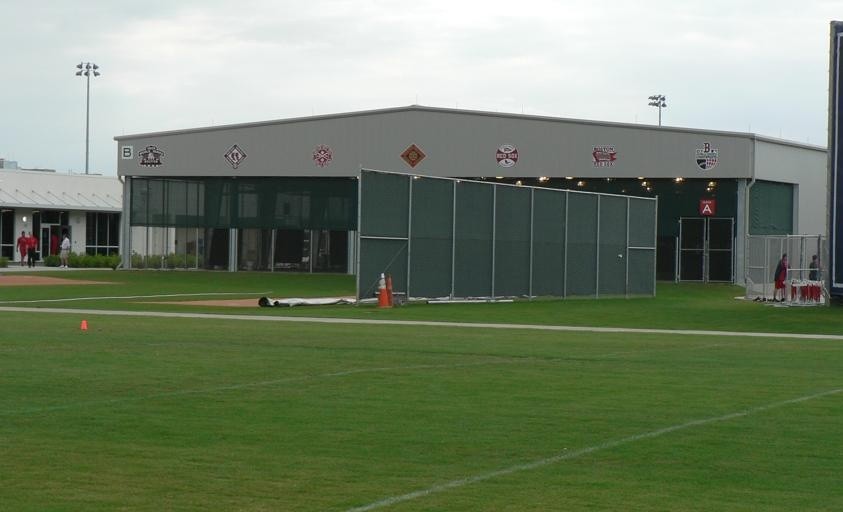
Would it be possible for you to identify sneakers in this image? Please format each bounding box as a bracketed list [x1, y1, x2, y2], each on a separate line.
[60, 265, 68, 269]
[772, 299, 780, 302]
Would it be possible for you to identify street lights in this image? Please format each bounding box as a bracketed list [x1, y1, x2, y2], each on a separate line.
[75, 62, 100, 174]
[648, 94, 666, 126]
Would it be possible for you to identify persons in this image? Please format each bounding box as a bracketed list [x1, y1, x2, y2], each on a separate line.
[15, 231, 27, 266]
[808, 255, 819, 280]
[24, 232, 37, 268]
[58, 234, 70, 269]
[773, 254, 792, 302]
[50, 232, 59, 256]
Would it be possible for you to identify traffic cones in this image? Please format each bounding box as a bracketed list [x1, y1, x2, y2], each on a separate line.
[376, 272, 394, 308]
